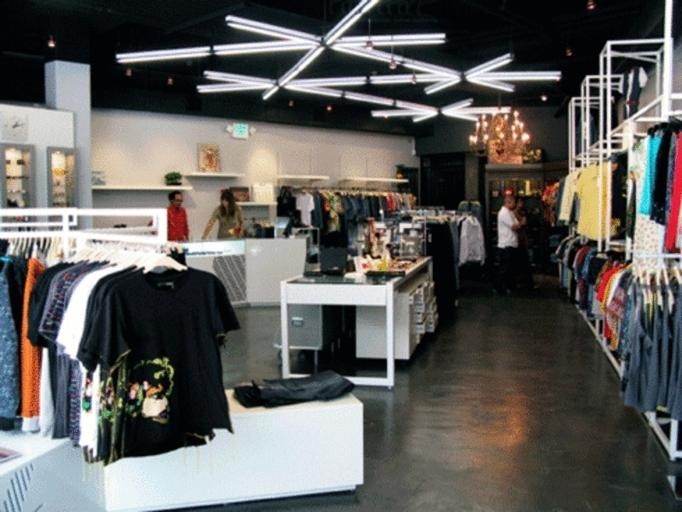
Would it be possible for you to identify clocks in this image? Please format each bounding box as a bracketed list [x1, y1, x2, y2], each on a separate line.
[232, 122, 249, 139]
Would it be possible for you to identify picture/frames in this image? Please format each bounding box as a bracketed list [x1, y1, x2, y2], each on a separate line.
[196, 142, 222, 174]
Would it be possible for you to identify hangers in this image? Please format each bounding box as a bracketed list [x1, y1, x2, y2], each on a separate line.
[2, 232, 188, 276]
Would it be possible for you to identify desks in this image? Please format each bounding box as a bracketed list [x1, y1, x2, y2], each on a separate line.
[278, 255, 433, 390]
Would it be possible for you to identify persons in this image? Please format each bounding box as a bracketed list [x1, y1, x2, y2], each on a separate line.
[492, 195, 538, 298]
[146, 191, 189, 242]
[511, 198, 536, 287]
[201, 189, 245, 241]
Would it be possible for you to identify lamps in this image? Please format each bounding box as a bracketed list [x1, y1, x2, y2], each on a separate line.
[468, 91, 542, 164]
[116, 0, 564, 124]
